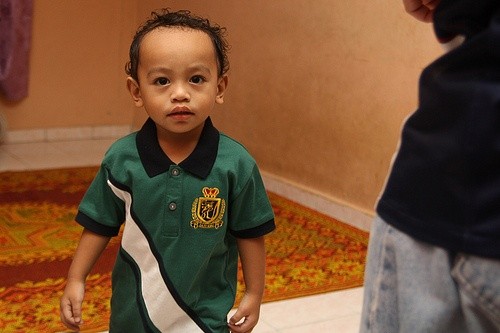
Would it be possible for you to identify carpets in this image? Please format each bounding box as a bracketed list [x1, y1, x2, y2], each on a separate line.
[0, 165, 370, 333]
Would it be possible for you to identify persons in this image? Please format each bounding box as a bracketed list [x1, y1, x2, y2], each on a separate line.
[60, 8, 277, 333]
[358, 0, 500, 333]
